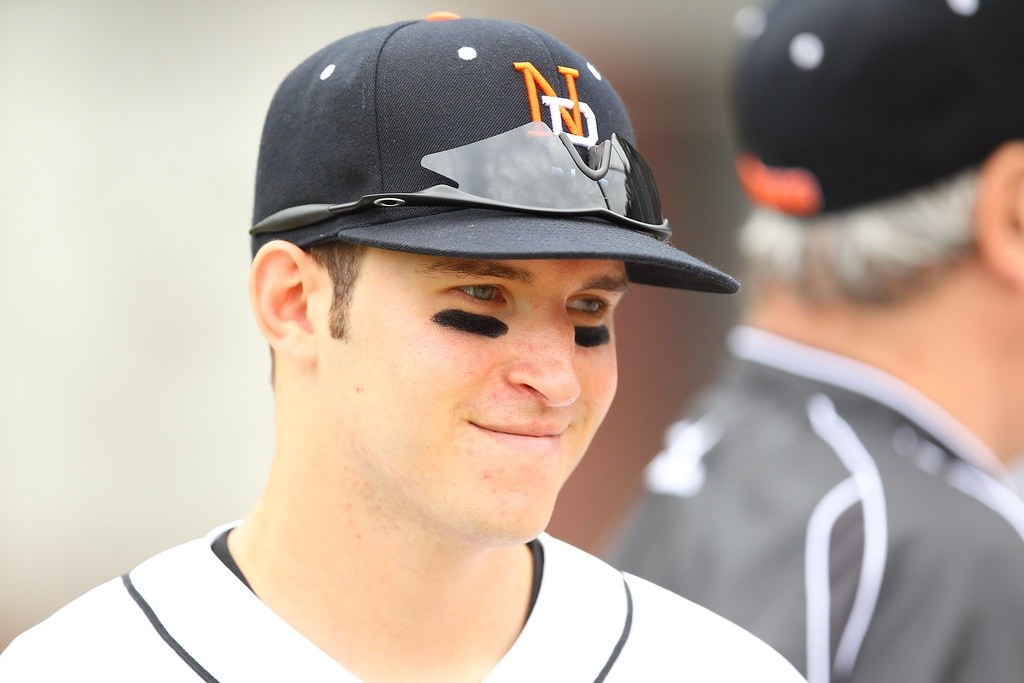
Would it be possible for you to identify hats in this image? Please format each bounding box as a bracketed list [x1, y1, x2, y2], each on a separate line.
[250, 11, 739, 293]
[729, 0, 1024, 221]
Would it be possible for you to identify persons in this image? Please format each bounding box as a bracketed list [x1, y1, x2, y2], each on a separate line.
[0, 13, 807, 683]
[607, 0, 1024, 683]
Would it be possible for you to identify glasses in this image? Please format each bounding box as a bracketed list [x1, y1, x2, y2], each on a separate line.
[249, 121, 673, 239]
